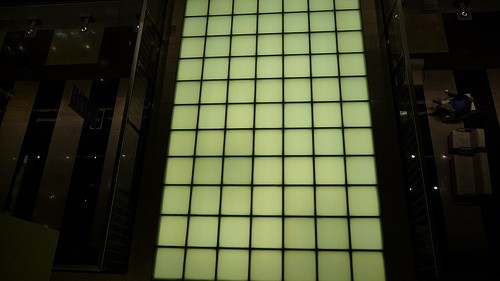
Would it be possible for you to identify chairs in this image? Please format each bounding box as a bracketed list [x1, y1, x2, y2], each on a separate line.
[403, 59, 425, 86]
[449, 128, 486, 153]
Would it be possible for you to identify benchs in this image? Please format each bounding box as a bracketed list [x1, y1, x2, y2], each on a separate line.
[451, 153, 492, 196]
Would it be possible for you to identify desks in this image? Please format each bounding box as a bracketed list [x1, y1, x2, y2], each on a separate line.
[392, 13, 448, 53]
